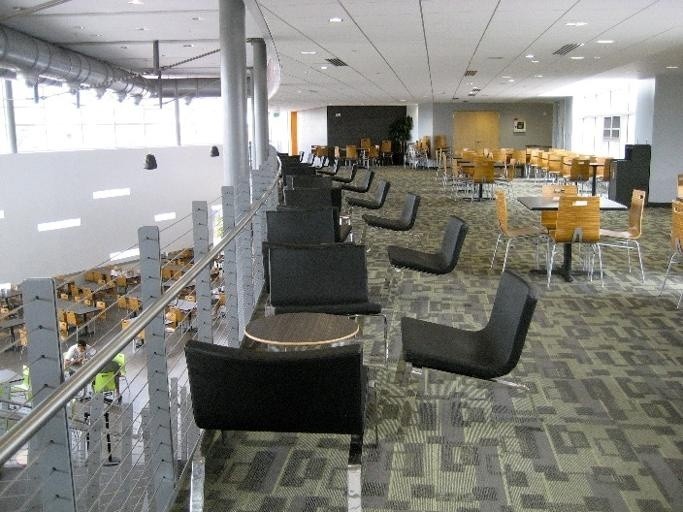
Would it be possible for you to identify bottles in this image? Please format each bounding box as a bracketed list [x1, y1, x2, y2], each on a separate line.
[552, 188, 562, 200]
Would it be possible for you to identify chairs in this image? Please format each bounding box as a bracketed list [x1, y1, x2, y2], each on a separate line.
[0, 249, 225, 404]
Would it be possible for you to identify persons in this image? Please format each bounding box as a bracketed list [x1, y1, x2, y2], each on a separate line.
[64, 338, 97, 366]
